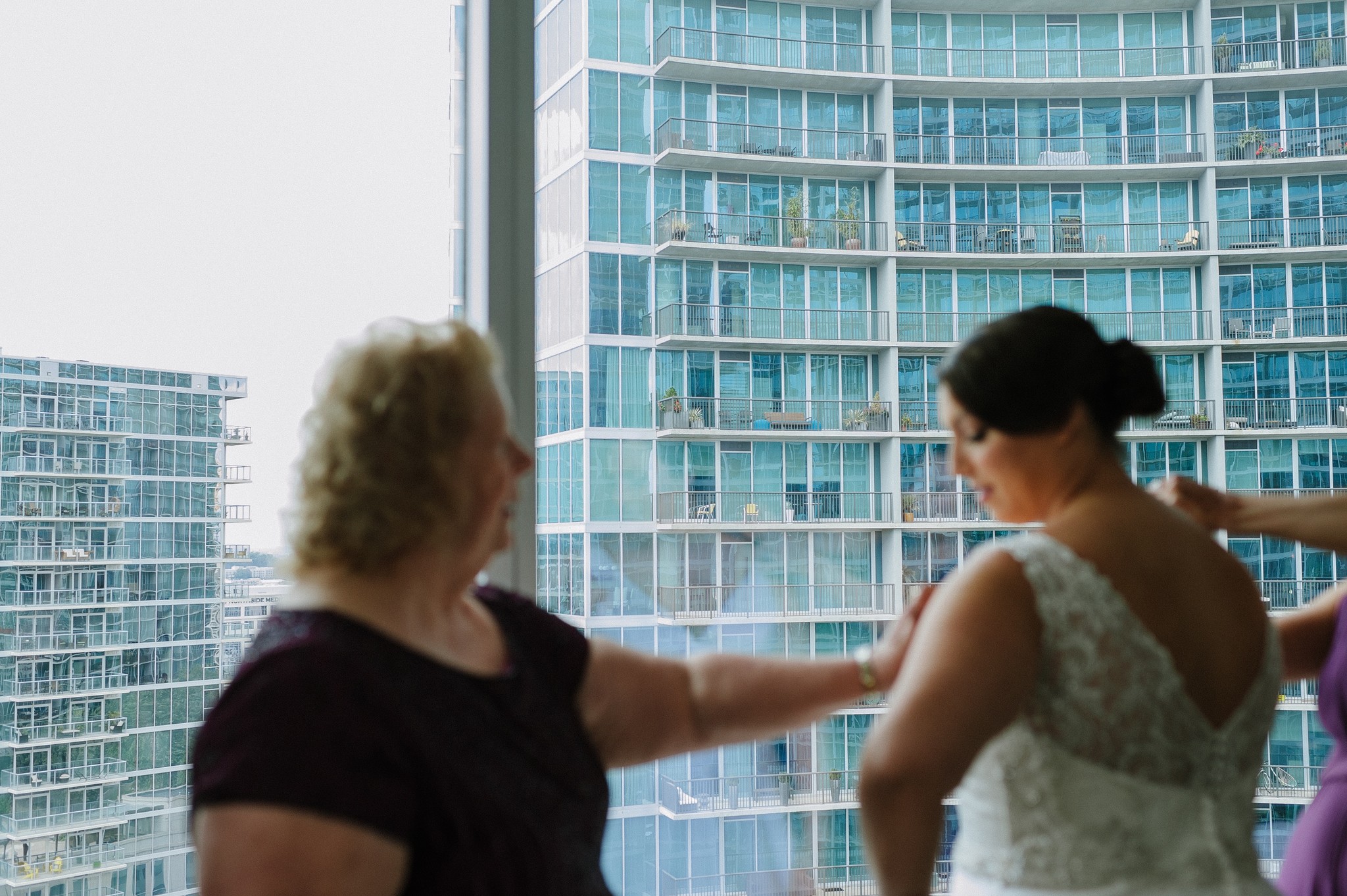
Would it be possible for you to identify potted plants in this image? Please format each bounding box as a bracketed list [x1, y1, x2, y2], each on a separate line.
[725, 768, 841, 809]
[786, 188, 862, 249]
[843, 390, 919, 522]
[232, 428, 249, 440]
[1061, 217, 1081, 243]
[660, 218, 695, 241]
[902, 494, 916, 521]
[1236, 125, 1267, 159]
[786, 502, 795, 522]
[688, 406, 706, 429]
[1190, 408, 1210, 429]
[1215, 32, 1332, 73]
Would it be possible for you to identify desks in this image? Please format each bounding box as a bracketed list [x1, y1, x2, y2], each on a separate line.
[918, 245, 928, 251]
[997, 230, 1014, 252]
[804, 503, 823, 524]
[1159, 243, 1173, 250]
[764, 149, 775, 155]
[22, 642, 32, 651]
[1304, 145, 1319, 156]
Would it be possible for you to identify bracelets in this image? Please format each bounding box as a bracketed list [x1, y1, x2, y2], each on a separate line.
[858, 646, 878, 696]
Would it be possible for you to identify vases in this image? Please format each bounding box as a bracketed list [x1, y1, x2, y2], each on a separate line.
[664, 412, 690, 429]
[1262, 154, 1271, 159]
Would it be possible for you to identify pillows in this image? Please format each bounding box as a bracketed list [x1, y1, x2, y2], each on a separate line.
[1158, 411, 1190, 420]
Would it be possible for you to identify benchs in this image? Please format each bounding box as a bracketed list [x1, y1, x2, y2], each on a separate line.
[663, 781, 697, 812]
[1227, 241, 1279, 249]
[764, 412, 812, 429]
[1151, 410, 1191, 429]
[1250, 422, 1297, 429]
[912, 423, 927, 432]
[1237, 60, 1276, 72]
[1226, 417, 1247, 430]
[753, 788, 794, 802]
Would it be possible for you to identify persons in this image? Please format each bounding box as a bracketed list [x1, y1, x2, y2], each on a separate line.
[854, 306, 1282, 896]
[189, 324, 933, 896]
[1160, 474, 1347, 896]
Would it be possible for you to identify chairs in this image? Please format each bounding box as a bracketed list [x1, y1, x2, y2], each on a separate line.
[718, 411, 754, 430]
[689, 503, 759, 523]
[1253, 331, 1272, 339]
[15, 461, 247, 878]
[703, 222, 763, 245]
[1227, 138, 1342, 339]
[739, 143, 795, 157]
[896, 226, 1199, 252]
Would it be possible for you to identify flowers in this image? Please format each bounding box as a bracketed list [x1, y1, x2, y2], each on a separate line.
[1256, 142, 1284, 154]
[657, 400, 681, 414]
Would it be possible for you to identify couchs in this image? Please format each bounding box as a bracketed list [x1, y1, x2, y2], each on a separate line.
[746, 870, 816, 896]
